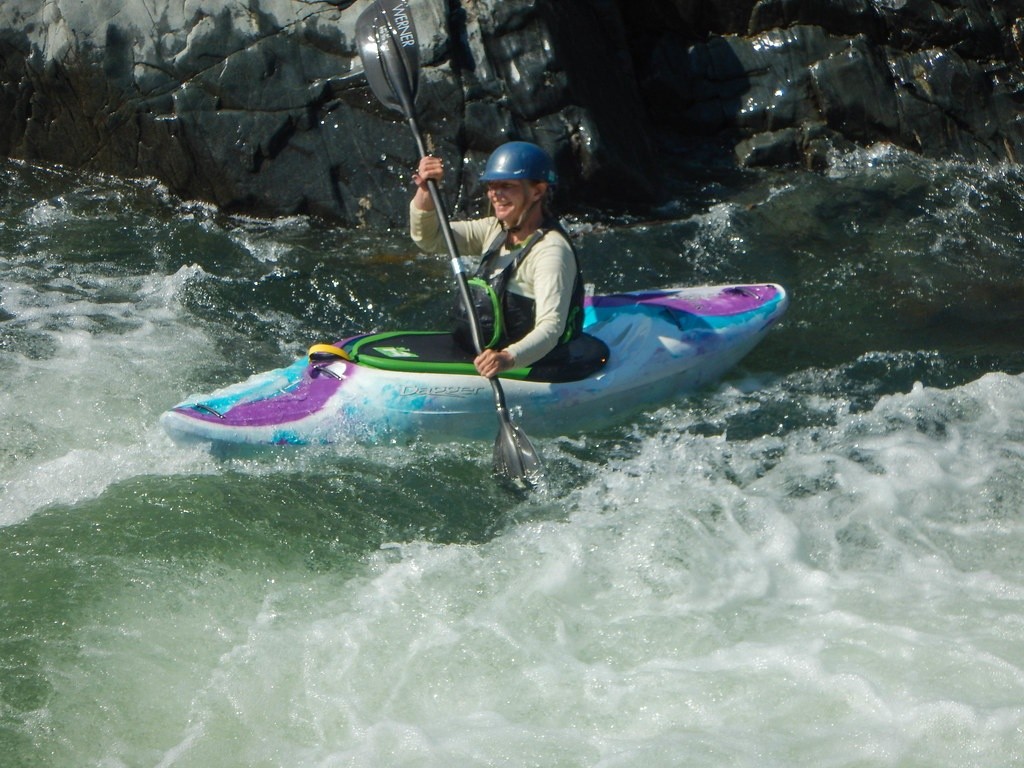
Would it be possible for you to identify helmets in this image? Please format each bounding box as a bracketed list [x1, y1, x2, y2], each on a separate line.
[478, 141, 556, 184]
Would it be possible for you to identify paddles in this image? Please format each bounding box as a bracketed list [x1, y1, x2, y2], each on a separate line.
[358, 0, 550, 501]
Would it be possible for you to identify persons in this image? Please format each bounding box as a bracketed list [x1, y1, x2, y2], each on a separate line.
[410, 142, 585, 378]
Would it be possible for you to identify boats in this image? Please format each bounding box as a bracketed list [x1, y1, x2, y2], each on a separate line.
[155, 284, 792, 457]
